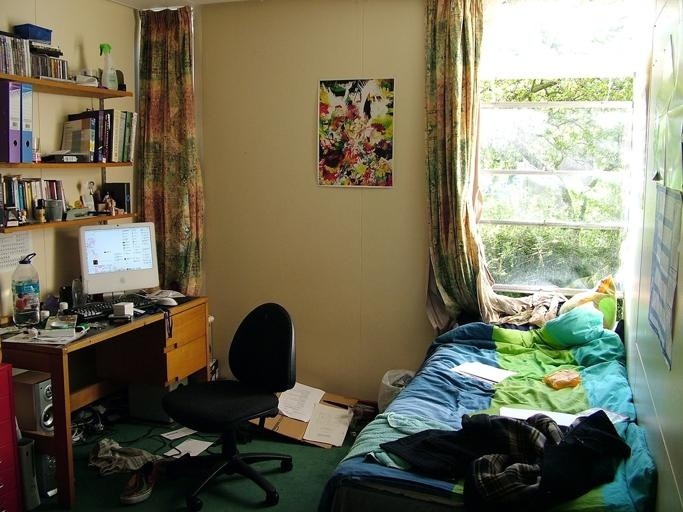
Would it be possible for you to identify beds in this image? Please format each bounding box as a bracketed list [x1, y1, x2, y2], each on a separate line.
[320, 293, 661, 512]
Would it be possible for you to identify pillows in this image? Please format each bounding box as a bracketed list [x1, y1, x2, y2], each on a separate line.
[558, 275, 617, 330]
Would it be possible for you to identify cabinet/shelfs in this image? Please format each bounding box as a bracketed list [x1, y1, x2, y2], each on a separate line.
[0, 75, 137, 233]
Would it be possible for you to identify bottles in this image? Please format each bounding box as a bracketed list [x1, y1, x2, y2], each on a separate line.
[35, 199, 47, 223]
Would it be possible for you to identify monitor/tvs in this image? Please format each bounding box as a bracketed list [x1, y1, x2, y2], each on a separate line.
[78, 222, 160, 299]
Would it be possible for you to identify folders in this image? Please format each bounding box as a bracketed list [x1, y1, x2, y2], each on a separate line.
[0, 81, 33, 164]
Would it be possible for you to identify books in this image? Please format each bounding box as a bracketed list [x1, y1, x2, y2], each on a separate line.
[0, 171, 67, 220]
[59, 109, 139, 163]
[101, 182, 127, 214]
[0, 33, 77, 83]
[44, 314, 78, 336]
[125, 182, 130, 214]
[40, 153, 89, 164]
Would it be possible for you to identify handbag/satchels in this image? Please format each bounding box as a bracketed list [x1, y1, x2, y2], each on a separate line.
[88, 437, 164, 477]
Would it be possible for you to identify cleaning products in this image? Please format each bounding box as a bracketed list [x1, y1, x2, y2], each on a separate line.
[100, 44, 119, 90]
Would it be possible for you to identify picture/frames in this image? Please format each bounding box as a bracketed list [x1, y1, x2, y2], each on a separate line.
[317, 78, 396, 189]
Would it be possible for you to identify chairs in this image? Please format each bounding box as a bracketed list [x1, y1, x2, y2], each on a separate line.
[163, 301, 296, 512]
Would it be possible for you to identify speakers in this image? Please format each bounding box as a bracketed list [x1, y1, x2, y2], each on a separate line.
[11, 369, 55, 433]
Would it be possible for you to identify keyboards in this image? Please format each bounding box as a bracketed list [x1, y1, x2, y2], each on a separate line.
[63, 294, 155, 321]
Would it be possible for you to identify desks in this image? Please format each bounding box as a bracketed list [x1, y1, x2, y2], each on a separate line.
[0, 297, 209, 512]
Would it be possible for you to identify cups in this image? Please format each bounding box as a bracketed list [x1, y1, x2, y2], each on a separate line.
[48, 199, 63, 221]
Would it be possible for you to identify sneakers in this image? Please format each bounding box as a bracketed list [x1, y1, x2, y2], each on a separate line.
[119, 461, 157, 505]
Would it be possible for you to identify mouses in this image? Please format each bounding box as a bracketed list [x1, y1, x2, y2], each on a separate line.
[156, 297, 178, 307]
